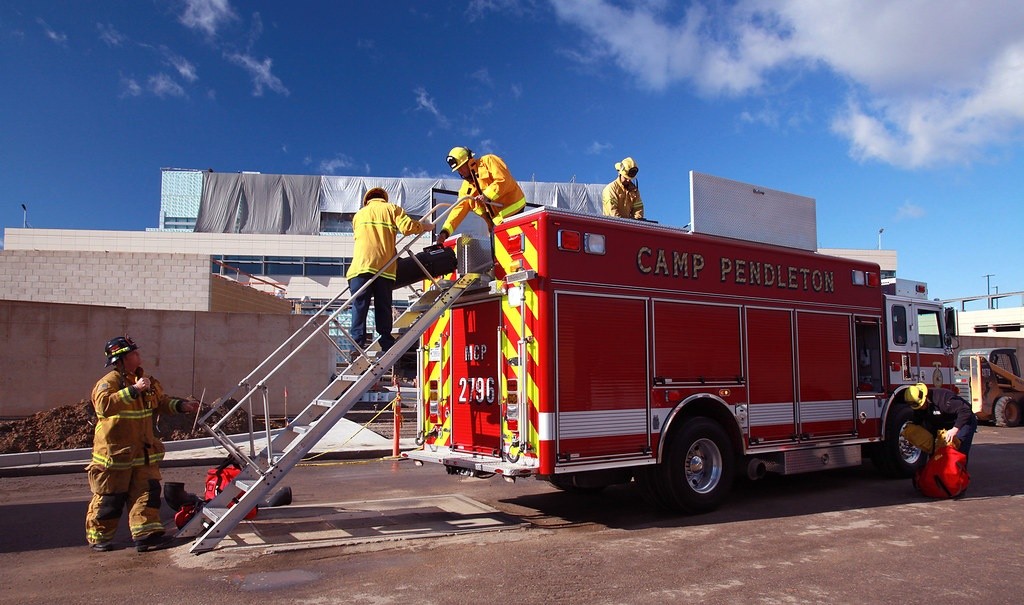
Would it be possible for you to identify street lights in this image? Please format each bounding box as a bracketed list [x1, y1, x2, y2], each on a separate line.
[982, 274, 995, 309]
[878, 227, 884, 250]
[21, 203, 27, 228]
[991, 286, 998, 309]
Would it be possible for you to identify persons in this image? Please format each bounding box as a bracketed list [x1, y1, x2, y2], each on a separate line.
[84, 337, 201, 552]
[348, 187, 436, 358]
[435, 145, 528, 226]
[603, 156, 644, 221]
[906, 384, 977, 497]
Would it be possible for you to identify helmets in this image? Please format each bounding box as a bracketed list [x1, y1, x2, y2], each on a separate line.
[614, 157, 638, 180]
[445, 146, 475, 173]
[103, 332, 142, 368]
[363, 187, 389, 206]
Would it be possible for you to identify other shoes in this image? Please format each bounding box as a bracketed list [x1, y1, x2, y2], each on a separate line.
[348, 350, 359, 363]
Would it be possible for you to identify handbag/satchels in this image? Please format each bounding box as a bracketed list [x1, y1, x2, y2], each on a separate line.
[918, 444, 970, 500]
[391, 243, 458, 290]
[204, 452, 258, 520]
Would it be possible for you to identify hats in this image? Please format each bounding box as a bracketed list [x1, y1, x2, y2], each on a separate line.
[905, 382, 928, 410]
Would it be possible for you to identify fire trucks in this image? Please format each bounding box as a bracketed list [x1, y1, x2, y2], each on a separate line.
[402, 171, 960, 517]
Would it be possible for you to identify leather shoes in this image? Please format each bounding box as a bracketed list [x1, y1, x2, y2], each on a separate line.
[134, 531, 173, 552]
[88, 541, 114, 551]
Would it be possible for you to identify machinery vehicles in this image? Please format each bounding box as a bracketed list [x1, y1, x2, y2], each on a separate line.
[955, 347, 1024, 427]
